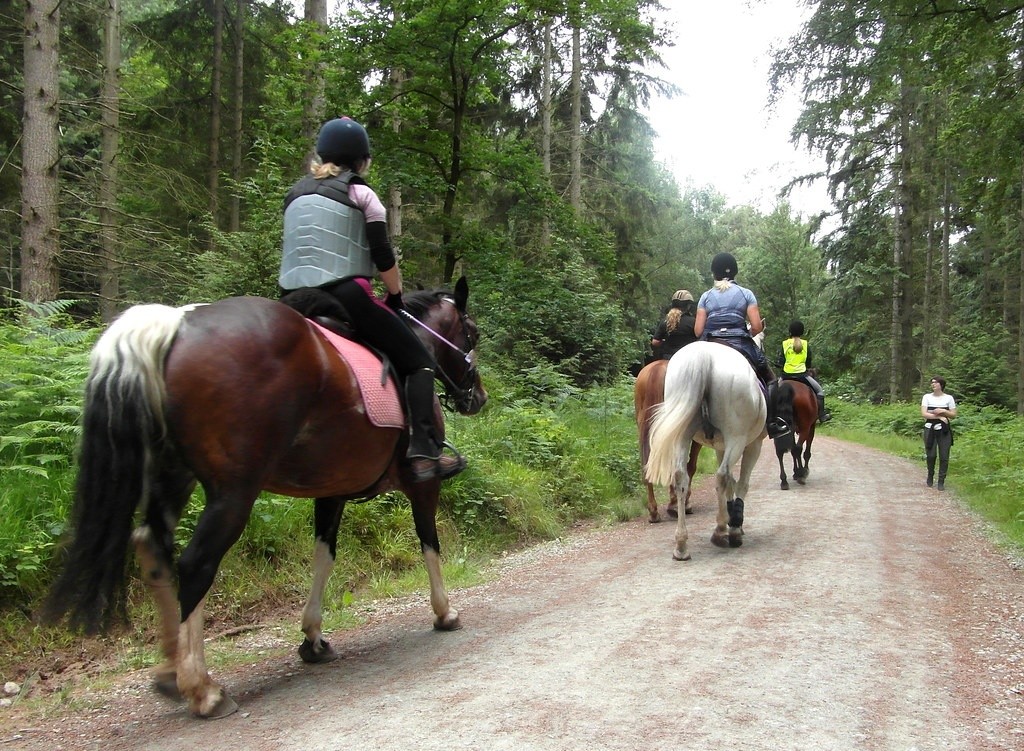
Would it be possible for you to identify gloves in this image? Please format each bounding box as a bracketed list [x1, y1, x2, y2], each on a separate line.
[384, 291, 405, 312]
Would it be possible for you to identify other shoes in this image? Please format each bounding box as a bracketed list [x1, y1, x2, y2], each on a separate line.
[927, 471, 935, 487]
[938, 479, 945, 490]
[410, 453, 467, 485]
[766, 423, 789, 439]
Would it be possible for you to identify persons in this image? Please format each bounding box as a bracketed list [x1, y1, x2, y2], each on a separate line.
[921, 376, 956, 490]
[280, 120, 470, 487]
[654, 291, 704, 364]
[694, 253, 788, 439]
[777, 320, 832, 425]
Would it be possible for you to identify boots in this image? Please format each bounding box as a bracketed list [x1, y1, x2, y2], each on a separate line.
[817, 395, 830, 423]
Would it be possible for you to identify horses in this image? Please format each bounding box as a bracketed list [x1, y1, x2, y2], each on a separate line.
[31, 275, 489, 720]
[641, 340, 769, 563]
[775, 380, 820, 492]
[634, 359, 705, 523]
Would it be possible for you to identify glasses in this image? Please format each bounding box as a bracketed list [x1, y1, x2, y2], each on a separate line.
[932, 381, 940, 383]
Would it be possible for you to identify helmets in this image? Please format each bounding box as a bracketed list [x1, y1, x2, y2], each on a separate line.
[711, 252, 739, 276]
[789, 322, 804, 335]
[316, 118, 370, 166]
[671, 289, 694, 304]
